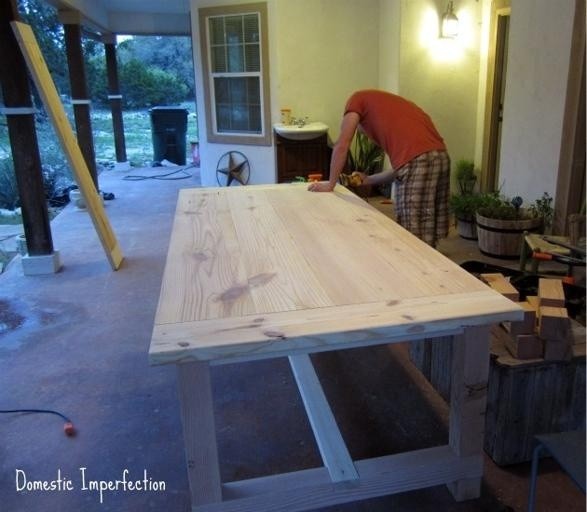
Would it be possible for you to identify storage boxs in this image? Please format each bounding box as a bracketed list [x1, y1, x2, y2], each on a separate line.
[423, 334, 586, 467]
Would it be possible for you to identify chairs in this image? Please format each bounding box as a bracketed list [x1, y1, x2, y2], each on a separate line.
[519, 200, 586, 277]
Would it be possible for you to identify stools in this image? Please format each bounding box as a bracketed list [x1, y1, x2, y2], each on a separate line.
[531, 429, 586, 512]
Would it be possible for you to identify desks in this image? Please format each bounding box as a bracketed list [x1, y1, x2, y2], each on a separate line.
[147, 180, 526, 512]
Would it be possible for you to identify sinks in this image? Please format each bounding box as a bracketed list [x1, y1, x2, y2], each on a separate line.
[273, 121, 329, 141]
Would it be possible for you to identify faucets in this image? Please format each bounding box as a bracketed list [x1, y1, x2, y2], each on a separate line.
[297, 119, 304, 128]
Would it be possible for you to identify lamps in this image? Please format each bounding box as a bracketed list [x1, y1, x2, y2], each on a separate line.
[438, 0, 460, 41]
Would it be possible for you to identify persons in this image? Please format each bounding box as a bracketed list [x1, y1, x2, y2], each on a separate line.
[307, 89, 451, 250]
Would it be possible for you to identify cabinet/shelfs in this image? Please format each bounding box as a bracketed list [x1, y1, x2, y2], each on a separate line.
[274, 134, 328, 183]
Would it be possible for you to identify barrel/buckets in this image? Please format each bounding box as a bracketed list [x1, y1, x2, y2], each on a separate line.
[457, 211, 478, 238]
[476, 207, 543, 259]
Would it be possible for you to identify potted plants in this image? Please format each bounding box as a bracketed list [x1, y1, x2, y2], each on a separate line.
[474, 191, 556, 260]
[450, 162, 502, 240]
